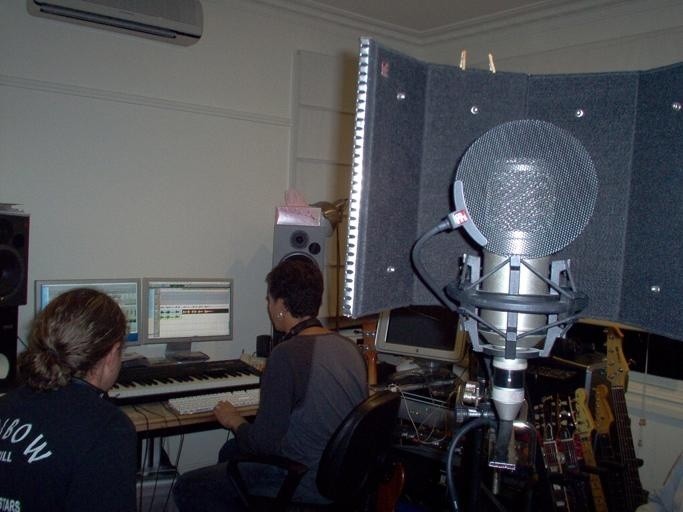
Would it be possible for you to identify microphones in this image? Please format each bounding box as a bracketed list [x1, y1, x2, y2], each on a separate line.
[453, 121, 598, 420]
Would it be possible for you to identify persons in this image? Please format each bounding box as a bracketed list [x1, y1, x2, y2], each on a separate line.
[0, 286, 142, 512]
[170, 253, 368, 509]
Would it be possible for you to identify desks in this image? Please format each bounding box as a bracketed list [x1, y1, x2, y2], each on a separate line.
[119, 392, 260, 484]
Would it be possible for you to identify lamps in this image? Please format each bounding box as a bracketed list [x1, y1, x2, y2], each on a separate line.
[308, 198, 350, 336]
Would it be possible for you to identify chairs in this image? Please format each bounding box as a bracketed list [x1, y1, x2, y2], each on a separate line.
[227, 390, 402, 512]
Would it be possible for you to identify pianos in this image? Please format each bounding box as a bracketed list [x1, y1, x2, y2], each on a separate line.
[106, 359, 262, 404]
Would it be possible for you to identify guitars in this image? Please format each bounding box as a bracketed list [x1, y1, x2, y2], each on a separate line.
[354, 320, 407, 511]
[533, 328, 649, 511]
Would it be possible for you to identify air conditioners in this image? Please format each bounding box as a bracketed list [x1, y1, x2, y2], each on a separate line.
[26, 0, 201, 48]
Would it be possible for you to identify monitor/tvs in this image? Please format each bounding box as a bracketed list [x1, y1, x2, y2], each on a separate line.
[374, 304, 463, 372]
[142, 277, 233, 363]
[35, 279, 148, 370]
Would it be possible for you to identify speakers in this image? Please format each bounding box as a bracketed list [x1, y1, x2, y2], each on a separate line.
[271, 223, 329, 347]
[0, 212, 30, 305]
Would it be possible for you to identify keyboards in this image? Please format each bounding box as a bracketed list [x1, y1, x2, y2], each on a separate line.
[168, 387, 262, 415]
